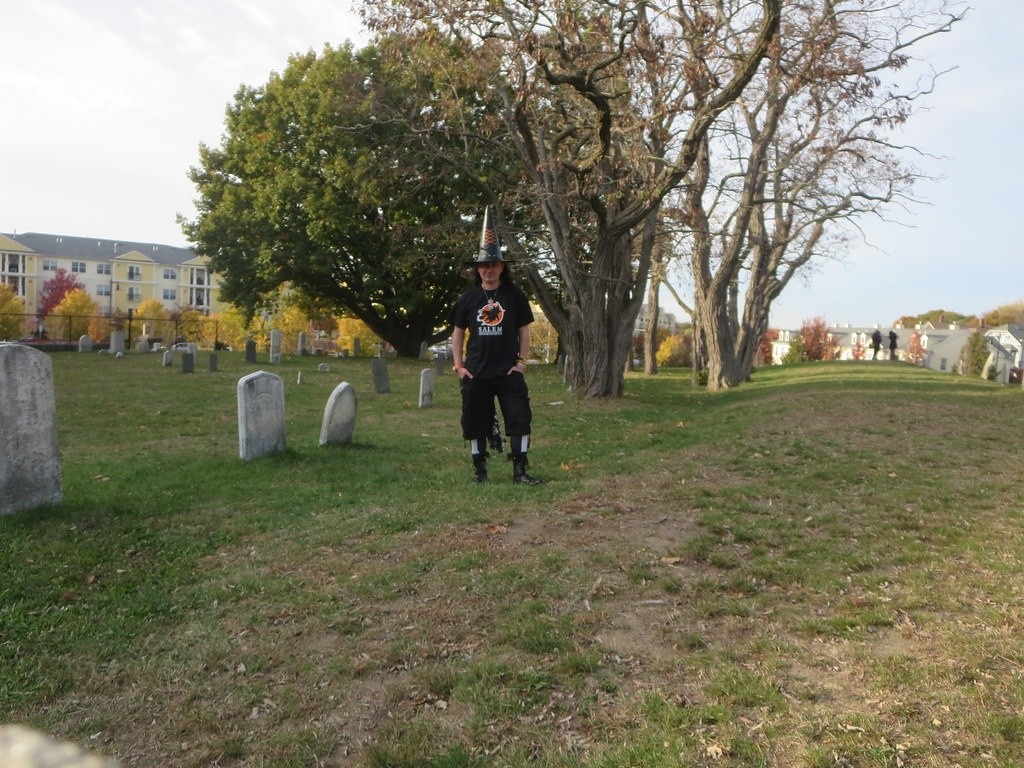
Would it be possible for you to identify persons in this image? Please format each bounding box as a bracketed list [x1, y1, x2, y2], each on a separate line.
[446, 261, 542, 486]
[889, 330, 898, 360]
[872, 330, 882, 360]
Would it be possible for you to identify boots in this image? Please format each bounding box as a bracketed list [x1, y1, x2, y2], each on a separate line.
[513, 453, 543, 485]
[471, 453, 487, 483]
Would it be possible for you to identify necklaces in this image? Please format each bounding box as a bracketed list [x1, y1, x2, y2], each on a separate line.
[482, 283, 498, 304]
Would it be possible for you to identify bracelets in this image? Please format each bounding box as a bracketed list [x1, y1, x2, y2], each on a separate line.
[516, 358, 527, 371]
[452, 365, 463, 373]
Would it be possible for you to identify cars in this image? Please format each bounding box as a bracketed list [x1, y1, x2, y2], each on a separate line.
[429, 345, 452, 360]
[171, 343, 189, 351]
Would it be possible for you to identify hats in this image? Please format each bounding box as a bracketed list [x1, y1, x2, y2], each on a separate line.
[462, 205, 512, 263]
